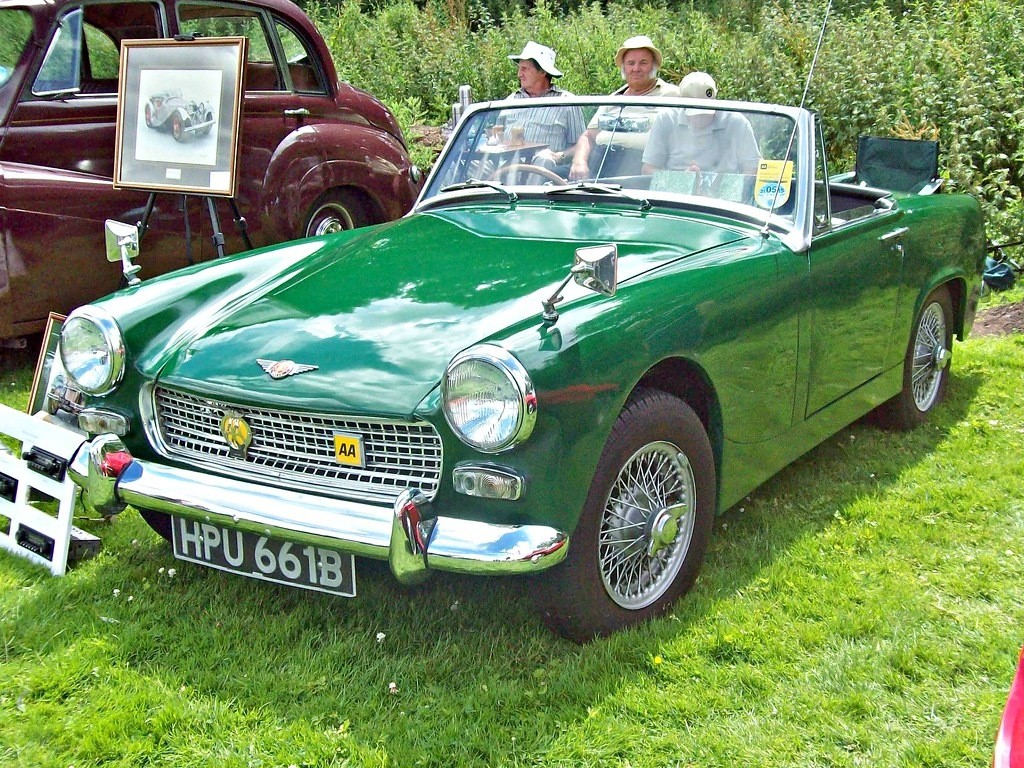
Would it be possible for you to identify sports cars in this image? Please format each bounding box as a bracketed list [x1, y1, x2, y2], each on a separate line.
[46, 93, 988, 647]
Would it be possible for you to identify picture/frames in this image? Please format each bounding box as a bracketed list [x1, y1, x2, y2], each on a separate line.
[27, 311, 68, 416]
[114, 35, 252, 198]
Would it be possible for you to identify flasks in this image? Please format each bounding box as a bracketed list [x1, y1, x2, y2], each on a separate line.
[452, 104, 464, 130]
[459, 85, 472, 115]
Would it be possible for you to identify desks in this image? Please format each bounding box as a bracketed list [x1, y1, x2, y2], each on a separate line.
[433, 139, 549, 185]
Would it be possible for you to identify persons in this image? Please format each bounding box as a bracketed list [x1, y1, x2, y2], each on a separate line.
[640, 72, 763, 174]
[442, 41, 587, 186]
[568, 36, 681, 190]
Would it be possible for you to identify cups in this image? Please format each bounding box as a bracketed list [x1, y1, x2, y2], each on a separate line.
[511, 127, 525, 146]
[493, 126, 503, 145]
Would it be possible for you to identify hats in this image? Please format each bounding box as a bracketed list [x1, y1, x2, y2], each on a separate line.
[614, 36, 662, 69]
[507, 40, 563, 79]
[679, 72, 716, 116]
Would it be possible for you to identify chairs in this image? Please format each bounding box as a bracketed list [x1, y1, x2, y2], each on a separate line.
[827, 135, 943, 198]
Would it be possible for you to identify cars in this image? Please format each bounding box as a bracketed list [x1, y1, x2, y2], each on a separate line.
[0, 0, 428, 348]
